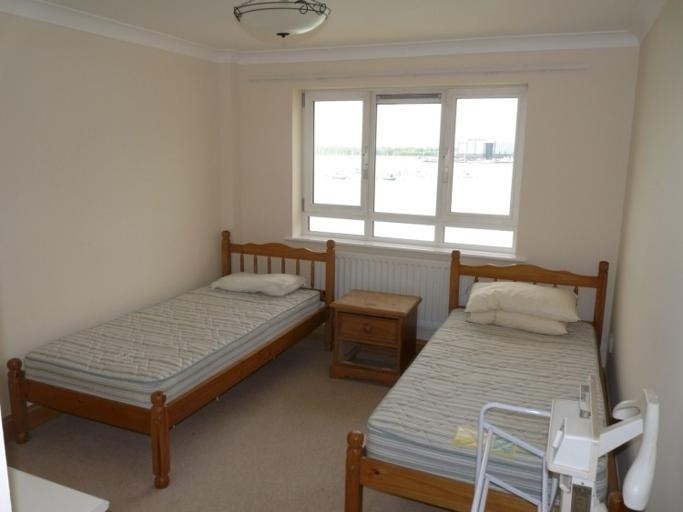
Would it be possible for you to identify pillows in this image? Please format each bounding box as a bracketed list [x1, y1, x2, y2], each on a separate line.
[209, 268, 303, 297]
[464, 281, 580, 323]
[470, 312, 566, 337]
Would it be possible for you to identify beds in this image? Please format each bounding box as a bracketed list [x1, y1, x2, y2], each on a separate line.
[6, 226, 335, 489]
[344, 250, 623, 512]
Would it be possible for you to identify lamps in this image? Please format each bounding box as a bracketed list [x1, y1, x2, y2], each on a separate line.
[222, 0, 330, 40]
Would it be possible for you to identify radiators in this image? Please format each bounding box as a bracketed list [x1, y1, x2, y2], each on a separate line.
[314, 248, 471, 331]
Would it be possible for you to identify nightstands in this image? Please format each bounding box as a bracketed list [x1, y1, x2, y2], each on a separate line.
[328, 290, 413, 390]
